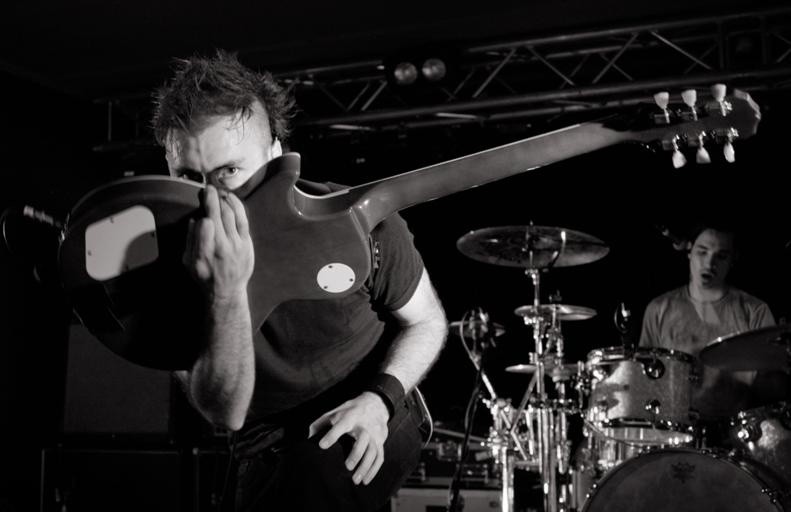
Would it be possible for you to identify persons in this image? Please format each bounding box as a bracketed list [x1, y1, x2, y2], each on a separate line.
[637, 221, 777, 412]
[148, 47, 450, 512]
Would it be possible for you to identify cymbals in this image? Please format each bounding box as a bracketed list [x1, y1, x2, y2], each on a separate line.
[457, 224, 611, 266]
[506, 363, 580, 375]
[513, 304, 596, 321]
[700, 324, 791, 371]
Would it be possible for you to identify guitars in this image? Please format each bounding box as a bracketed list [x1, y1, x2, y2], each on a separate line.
[57, 83, 762, 371]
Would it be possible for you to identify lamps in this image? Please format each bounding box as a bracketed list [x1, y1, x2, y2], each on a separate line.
[386, 43, 459, 91]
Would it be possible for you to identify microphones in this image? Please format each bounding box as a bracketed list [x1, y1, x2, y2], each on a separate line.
[469, 311, 481, 355]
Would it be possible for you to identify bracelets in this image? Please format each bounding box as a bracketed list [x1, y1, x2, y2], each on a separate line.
[362, 370, 408, 422]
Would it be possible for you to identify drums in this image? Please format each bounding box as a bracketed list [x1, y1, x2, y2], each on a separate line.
[727, 400, 791, 489]
[579, 445, 791, 512]
[580, 429, 653, 471]
[574, 345, 703, 451]
